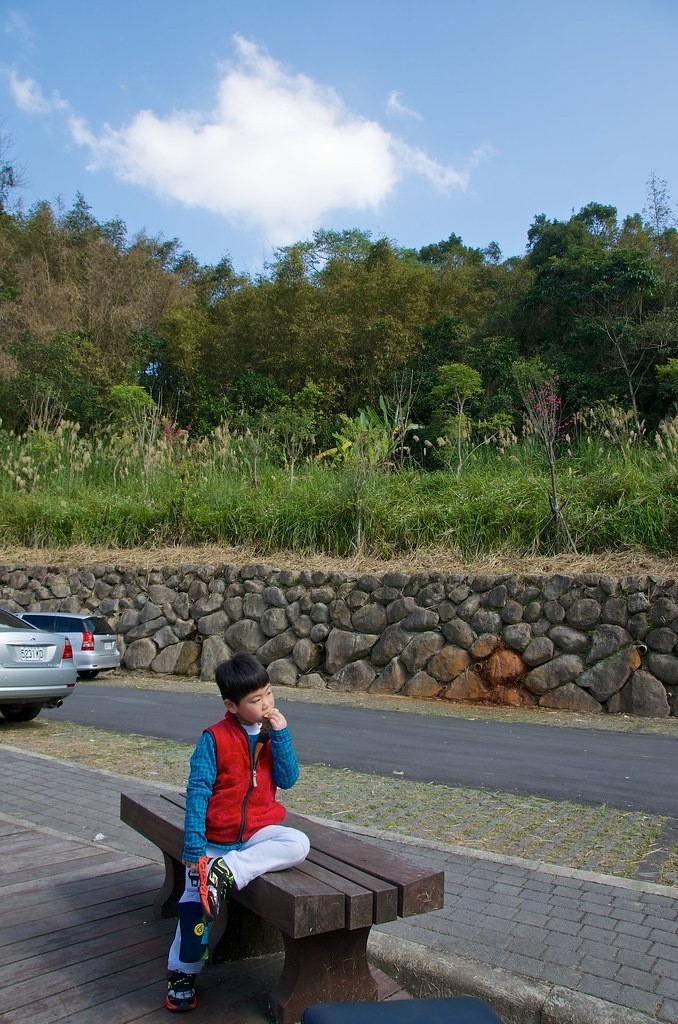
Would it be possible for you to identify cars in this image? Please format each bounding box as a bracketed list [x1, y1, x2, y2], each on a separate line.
[0, 606, 76, 722]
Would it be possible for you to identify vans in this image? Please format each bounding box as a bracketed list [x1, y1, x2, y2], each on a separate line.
[11, 613, 121, 679]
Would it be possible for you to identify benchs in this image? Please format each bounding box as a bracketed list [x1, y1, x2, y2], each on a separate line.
[119, 792, 445, 1024]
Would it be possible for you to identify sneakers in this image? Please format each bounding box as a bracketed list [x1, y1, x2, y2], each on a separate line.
[198, 856, 234, 922]
[166, 969, 197, 1011]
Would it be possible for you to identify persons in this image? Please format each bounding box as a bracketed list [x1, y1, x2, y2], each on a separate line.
[164, 654, 310, 1011]
